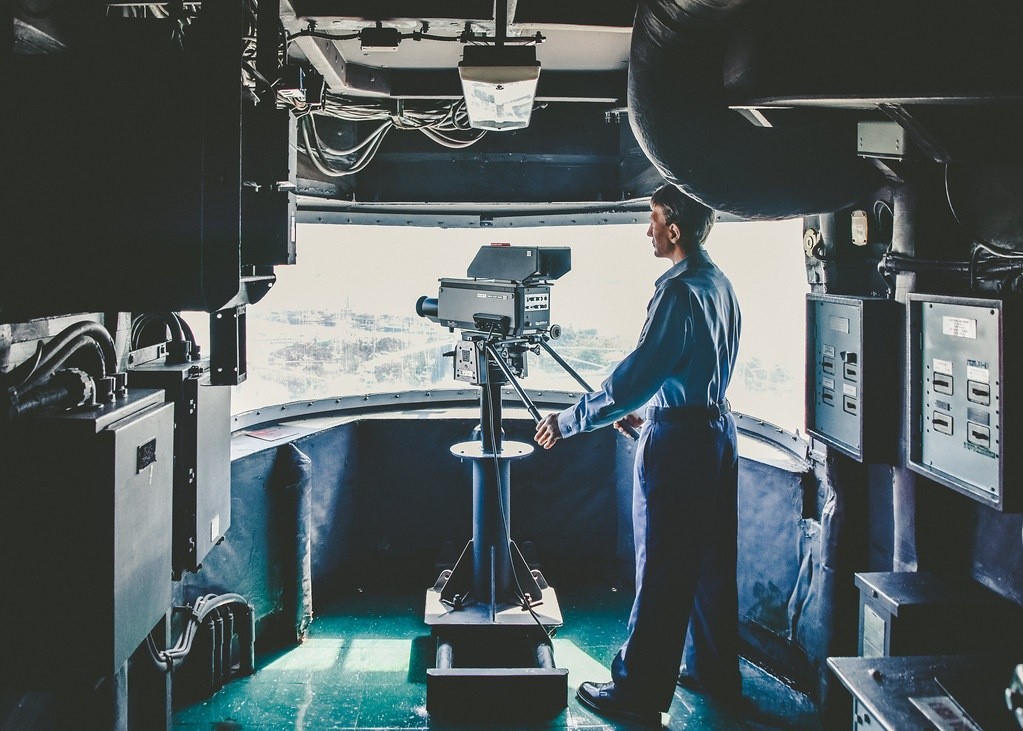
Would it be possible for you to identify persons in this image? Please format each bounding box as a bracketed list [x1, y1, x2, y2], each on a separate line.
[535, 183, 746, 726]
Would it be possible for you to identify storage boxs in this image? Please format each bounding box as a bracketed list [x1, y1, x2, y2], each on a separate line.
[458, 0, 542, 132]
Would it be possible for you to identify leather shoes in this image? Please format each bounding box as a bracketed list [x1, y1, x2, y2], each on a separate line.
[577, 681, 662, 727]
[676, 673, 737, 700]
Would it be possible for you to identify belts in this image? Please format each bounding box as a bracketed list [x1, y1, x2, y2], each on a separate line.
[649, 401, 729, 421]
